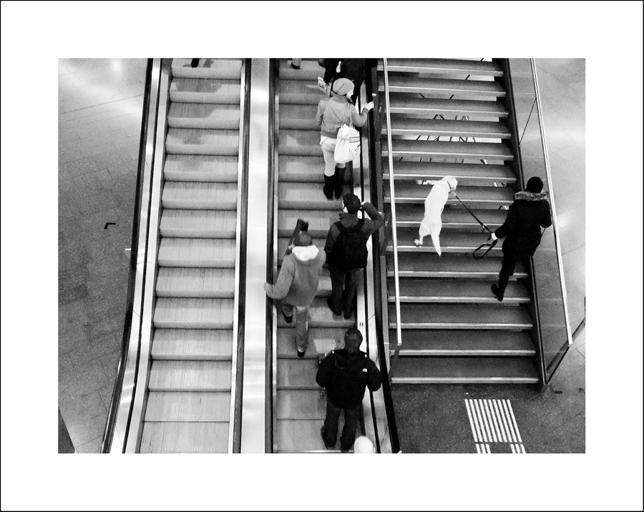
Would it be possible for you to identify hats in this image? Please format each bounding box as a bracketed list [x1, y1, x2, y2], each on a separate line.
[332, 77, 355, 96]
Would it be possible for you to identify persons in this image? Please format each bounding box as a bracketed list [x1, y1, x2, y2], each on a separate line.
[264, 231, 327, 358]
[322, 58, 380, 105]
[315, 78, 374, 200]
[490, 177, 553, 301]
[325, 192, 386, 319]
[314, 326, 382, 452]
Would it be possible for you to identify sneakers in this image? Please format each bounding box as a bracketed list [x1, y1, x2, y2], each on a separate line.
[326, 294, 355, 321]
[294, 336, 305, 359]
[340, 437, 350, 452]
[491, 283, 503, 301]
[322, 427, 337, 449]
[281, 308, 294, 325]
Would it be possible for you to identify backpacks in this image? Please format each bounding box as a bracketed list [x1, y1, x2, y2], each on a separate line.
[332, 219, 368, 272]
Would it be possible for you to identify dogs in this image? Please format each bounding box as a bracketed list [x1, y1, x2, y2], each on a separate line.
[413, 175, 458, 257]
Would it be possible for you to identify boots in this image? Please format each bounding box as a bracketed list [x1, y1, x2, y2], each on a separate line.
[334, 167, 346, 200]
[323, 173, 335, 201]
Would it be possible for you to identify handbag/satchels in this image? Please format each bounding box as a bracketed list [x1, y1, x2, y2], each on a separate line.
[333, 105, 361, 164]
